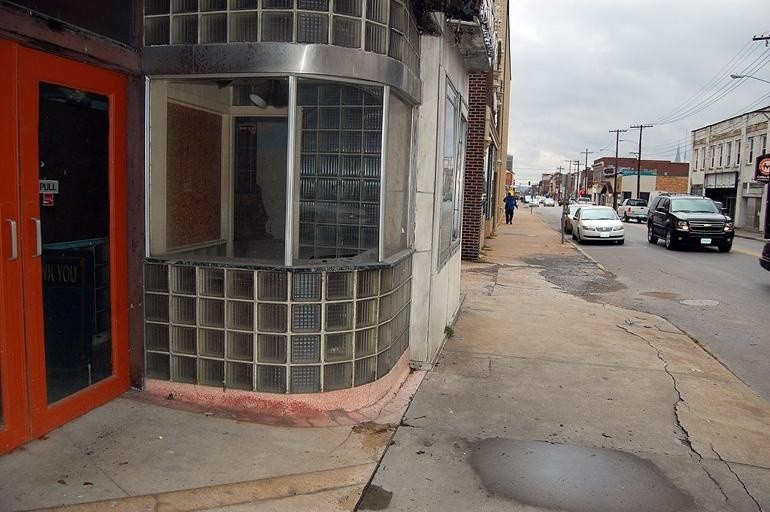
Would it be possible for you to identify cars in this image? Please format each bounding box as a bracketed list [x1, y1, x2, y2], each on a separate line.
[519, 193, 590, 208]
[570, 205, 625, 247]
[559, 202, 594, 234]
[758, 242, 770, 272]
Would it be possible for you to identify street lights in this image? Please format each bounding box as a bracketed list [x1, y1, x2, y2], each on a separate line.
[599, 148, 618, 209]
[619, 139, 643, 199]
[729, 72, 770, 84]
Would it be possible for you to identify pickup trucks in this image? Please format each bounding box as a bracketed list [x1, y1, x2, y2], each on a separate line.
[617, 197, 650, 223]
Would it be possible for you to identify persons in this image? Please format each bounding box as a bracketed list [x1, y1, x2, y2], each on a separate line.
[503, 192, 519, 224]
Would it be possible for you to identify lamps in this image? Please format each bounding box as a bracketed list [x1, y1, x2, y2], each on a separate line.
[244, 78, 287, 109]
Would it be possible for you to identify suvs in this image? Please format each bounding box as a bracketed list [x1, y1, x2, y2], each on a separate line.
[646, 191, 736, 254]
